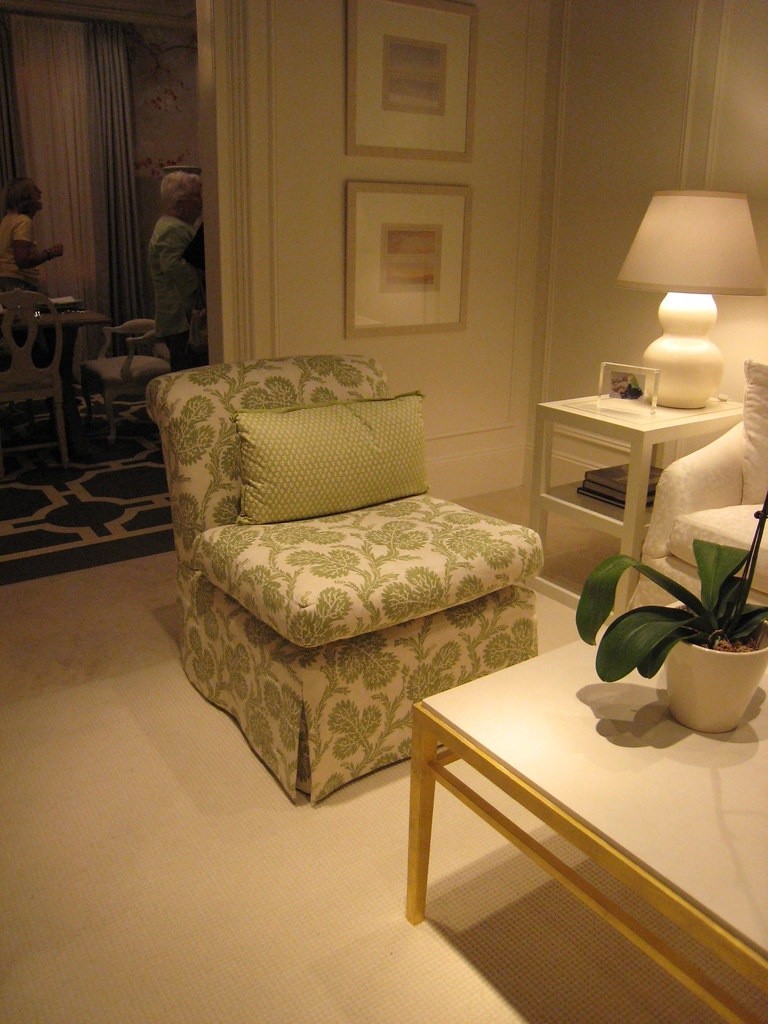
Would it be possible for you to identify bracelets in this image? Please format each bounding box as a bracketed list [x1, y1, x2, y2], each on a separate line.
[45, 249, 52, 260]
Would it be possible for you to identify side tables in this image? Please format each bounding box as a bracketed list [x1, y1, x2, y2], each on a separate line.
[525, 392, 744, 626]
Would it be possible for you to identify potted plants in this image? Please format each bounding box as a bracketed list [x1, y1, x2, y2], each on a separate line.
[575, 540, 768, 733]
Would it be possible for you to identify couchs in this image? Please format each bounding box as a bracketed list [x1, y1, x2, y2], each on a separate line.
[146, 353, 544, 809]
[631, 422, 768, 609]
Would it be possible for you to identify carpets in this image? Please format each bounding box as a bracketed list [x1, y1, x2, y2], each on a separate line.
[0, 393, 175, 587]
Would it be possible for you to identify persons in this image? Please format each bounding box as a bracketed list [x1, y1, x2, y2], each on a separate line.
[0, 177, 82, 407]
[148, 171, 206, 456]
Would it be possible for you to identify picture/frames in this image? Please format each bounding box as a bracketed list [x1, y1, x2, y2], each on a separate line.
[346, 0, 477, 164]
[597, 362, 660, 416]
[344, 181, 472, 340]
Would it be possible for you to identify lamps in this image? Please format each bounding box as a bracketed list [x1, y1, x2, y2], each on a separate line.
[614, 189, 768, 409]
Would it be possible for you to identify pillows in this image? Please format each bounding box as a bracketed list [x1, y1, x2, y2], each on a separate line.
[741, 359, 768, 505]
[228, 387, 428, 525]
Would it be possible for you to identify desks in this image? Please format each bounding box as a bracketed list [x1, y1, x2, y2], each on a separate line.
[405, 626, 768, 1024]
[15, 306, 112, 444]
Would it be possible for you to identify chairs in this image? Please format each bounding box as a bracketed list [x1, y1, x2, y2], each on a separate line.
[0, 289, 72, 474]
[81, 319, 174, 444]
